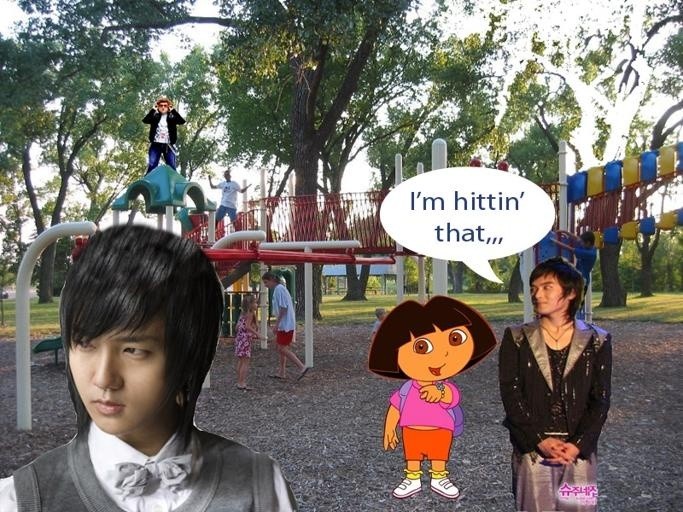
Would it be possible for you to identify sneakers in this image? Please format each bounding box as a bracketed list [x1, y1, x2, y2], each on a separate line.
[430, 477, 460, 499]
[392, 477, 422, 498]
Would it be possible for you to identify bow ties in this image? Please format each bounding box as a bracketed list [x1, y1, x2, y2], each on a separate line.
[115, 453, 192, 497]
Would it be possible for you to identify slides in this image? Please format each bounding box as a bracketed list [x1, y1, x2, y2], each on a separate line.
[33, 336, 62, 353]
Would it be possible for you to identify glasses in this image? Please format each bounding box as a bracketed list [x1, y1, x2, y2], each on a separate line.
[159, 105, 167, 107]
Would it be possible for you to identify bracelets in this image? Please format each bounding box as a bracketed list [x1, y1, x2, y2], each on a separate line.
[436, 381, 444, 399]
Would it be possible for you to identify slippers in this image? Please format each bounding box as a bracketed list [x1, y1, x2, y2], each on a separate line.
[296, 367, 308, 381]
[267, 373, 286, 380]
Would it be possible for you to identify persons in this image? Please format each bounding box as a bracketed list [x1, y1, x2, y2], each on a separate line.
[550, 230, 597, 294]
[142, 98, 185, 177]
[208, 170, 252, 228]
[368, 296, 496, 499]
[235, 295, 260, 391]
[1, 224, 299, 512]
[370, 308, 385, 341]
[499, 255, 613, 512]
[262, 272, 308, 381]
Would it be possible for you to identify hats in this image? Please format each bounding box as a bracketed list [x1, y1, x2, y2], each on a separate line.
[156, 95, 172, 105]
